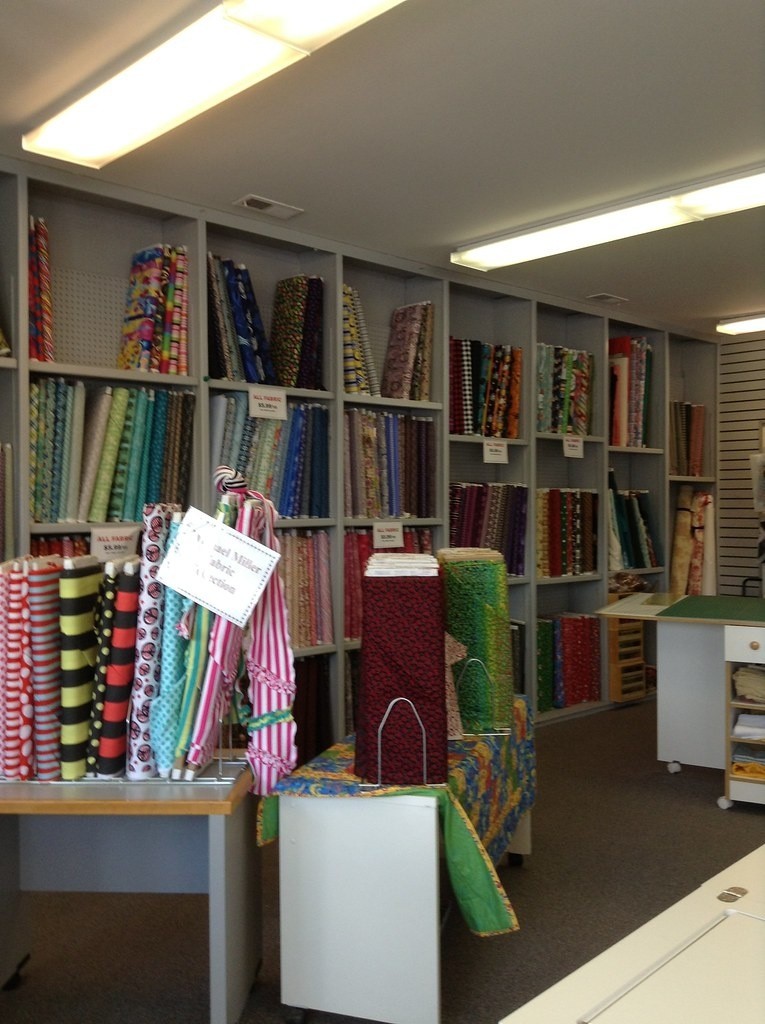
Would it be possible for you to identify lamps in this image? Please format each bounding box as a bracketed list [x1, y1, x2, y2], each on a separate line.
[449, 160, 765, 273]
[716, 314, 765, 336]
[21, 1, 409, 169]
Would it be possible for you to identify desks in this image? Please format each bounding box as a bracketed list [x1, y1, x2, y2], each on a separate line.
[2, 747, 258, 1024]
[594, 591, 765, 773]
[258, 693, 535, 1024]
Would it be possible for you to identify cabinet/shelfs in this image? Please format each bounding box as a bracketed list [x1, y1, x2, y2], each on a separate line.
[443, 269, 721, 729]
[0, 153, 443, 780]
[717, 625, 765, 809]
